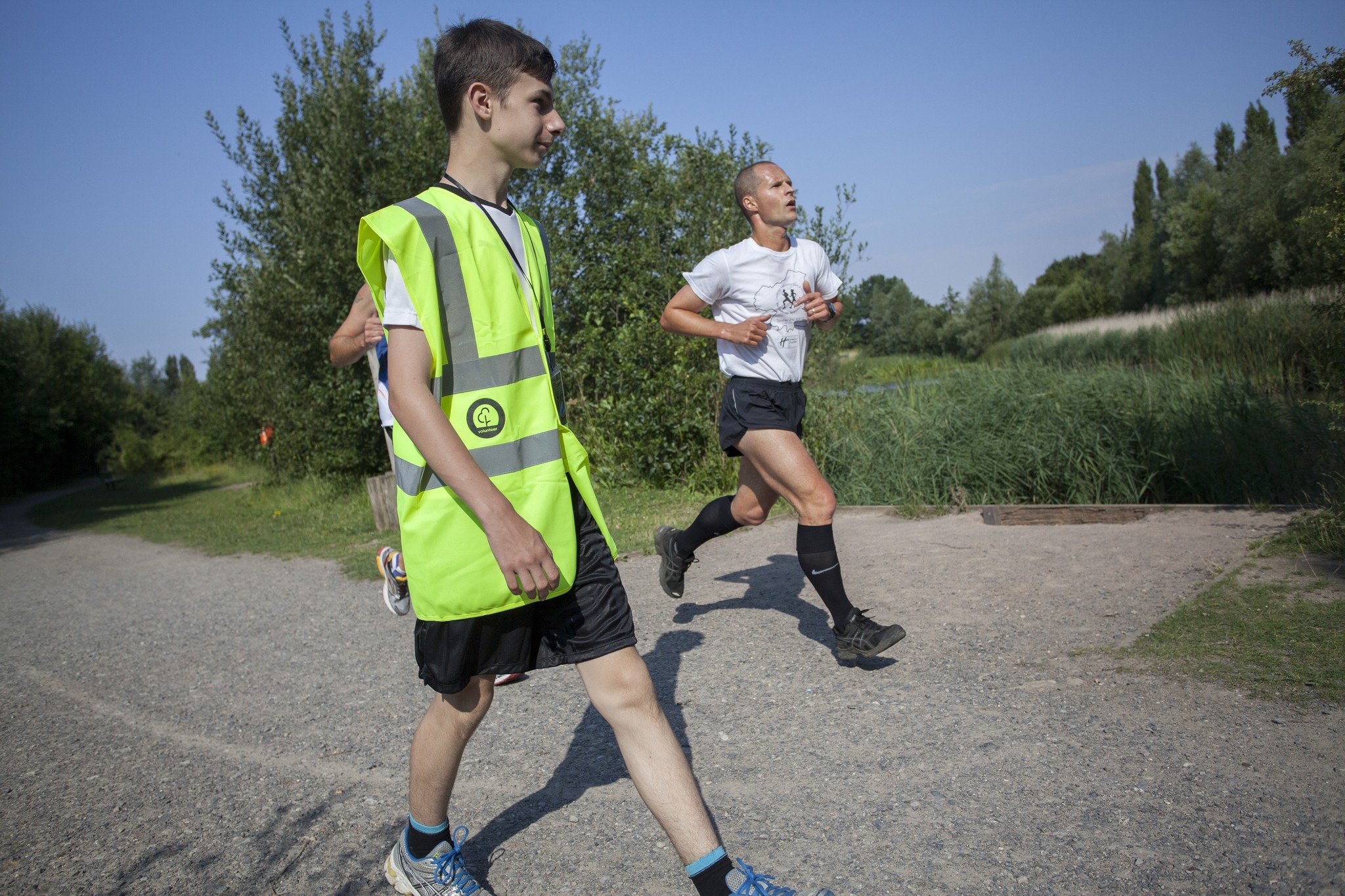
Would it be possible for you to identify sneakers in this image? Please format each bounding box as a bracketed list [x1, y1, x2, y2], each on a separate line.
[383, 826, 492, 895]
[833, 608, 906, 660]
[726, 858, 834, 896]
[494, 673, 523, 686]
[375, 546, 410, 616]
[654, 526, 700, 599]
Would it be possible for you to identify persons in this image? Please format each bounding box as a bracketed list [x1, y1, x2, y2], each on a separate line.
[356, 17, 799, 896]
[654, 160, 905, 659]
[329, 281, 526, 688]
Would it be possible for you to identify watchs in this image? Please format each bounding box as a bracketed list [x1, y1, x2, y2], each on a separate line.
[823, 301, 836, 322]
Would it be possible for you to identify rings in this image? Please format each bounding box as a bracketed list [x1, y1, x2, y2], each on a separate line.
[812, 308, 815, 314]
